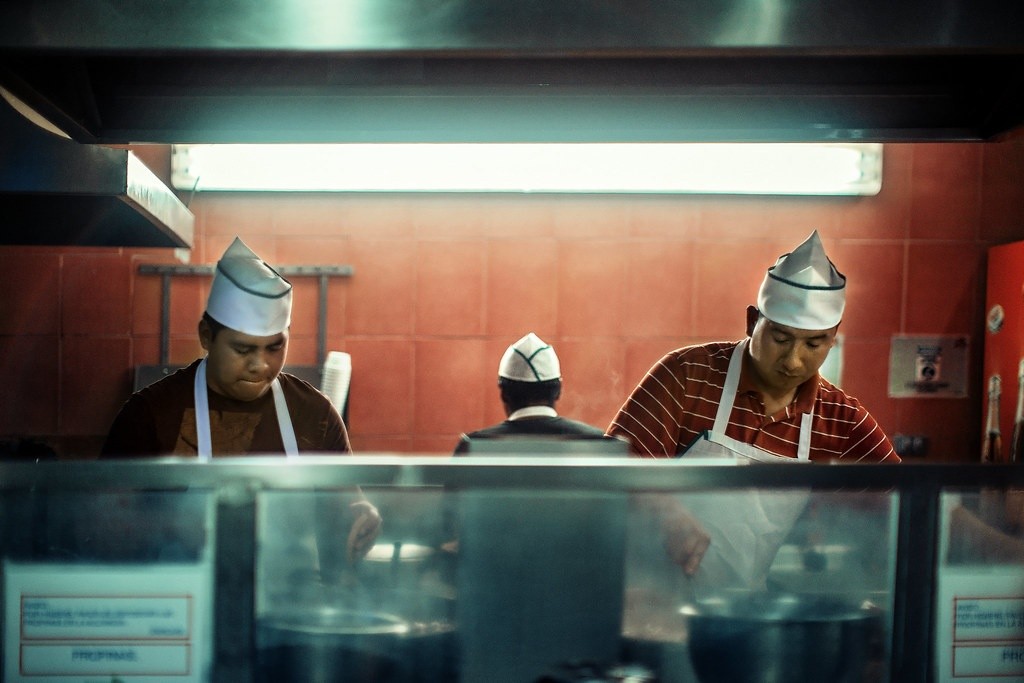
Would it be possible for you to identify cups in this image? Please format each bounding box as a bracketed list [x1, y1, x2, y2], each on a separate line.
[321, 351, 353, 416]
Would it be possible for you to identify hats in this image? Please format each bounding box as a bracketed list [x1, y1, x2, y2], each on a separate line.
[758, 229, 848, 330]
[206, 237, 293, 337]
[498, 332, 562, 384]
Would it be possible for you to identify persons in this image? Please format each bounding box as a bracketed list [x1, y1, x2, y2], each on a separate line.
[603, 229, 1024, 602]
[442, 333, 619, 572]
[101, 236, 384, 585]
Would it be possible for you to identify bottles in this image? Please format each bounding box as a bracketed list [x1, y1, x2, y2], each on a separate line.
[1009, 359, 1024, 465]
[982, 375, 1003, 464]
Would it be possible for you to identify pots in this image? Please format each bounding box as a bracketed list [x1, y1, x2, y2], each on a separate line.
[680, 599, 875, 683]
[257, 609, 409, 645]
